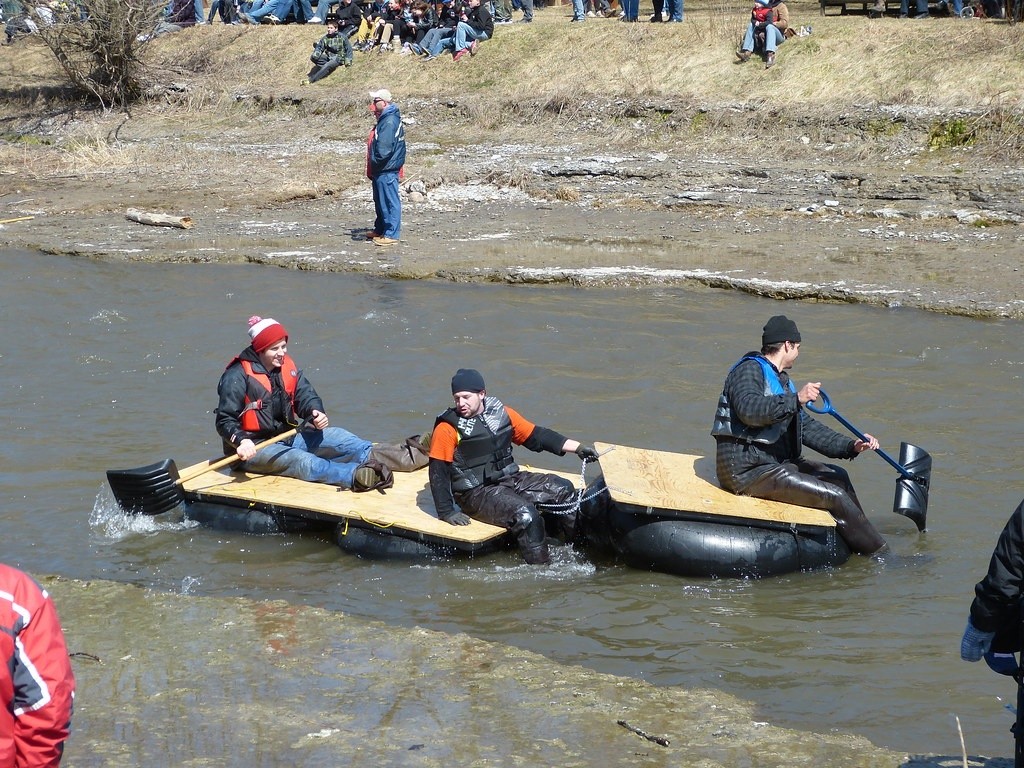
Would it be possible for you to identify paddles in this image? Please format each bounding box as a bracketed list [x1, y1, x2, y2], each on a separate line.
[805, 388, 934, 530]
[105, 420, 319, 517]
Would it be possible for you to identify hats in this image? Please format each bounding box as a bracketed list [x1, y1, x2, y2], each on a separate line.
[754, 0, 769, 7]
[369, 89, 392, 101]
[248, 316, 288, 352]
[451, 369, 486, 395]
[762, 315, 801, 345]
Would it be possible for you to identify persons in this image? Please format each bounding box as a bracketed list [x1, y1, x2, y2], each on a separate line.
[0, 0, 86, 44]
[586, 0, 616, 18]
[215, 315, 432, 490]
[300, 22, 353, 86]
[571, 0, 584, 22]
[735, 0, 789, 68]
[712, 316, 890, 556]
[0, 563, 75, 768]
[620, 0, 639, 21]
[961, 500, 1024, 663]
[135, 0, 544, 62]
[366, 89, 406, 246]
[869, 0, 1002, 18]
[651, 0, 684, 23]
[429, 369, 600, 565]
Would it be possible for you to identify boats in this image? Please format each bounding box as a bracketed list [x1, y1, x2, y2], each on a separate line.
[177, 442, 587, 566]
[575, 439, 852, 580]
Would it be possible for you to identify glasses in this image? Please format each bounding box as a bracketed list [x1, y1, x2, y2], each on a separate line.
[373, 99, 383, 103]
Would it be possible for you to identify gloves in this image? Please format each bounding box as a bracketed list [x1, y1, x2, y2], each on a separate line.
[447, 512, 471, 526]
[575, 443, 599, 463]
[961, 615, 995, 662]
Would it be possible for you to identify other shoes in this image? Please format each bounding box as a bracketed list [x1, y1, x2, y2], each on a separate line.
[766, 53, 774, 68]
[453, 39, 479, 61]
[351, 40, 375, 53]
[418, 431, 432, 451]
[356, 467, 382, 487]
[237, 11, 260, 26]
[586, 9, 615, 18]
[493, 19, 512, 25]
[736, 51, 751, 62]
[264, 15, 278, 25]
[308, 16, 321, 24]
[409, 43, 431, 57]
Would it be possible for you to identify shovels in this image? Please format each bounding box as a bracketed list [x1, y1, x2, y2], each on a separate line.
[104, 416, 317, 516]
[806, 389, 933, 532]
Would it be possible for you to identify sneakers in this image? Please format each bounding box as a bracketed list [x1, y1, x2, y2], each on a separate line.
[372, 237, 400, 246]
[366, 231, 379, 238]
